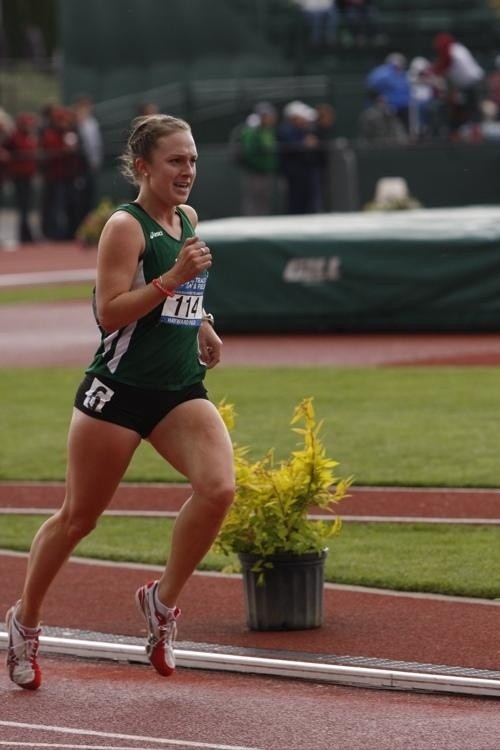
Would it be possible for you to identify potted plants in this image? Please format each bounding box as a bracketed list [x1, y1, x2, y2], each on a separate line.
[207, 395, 358, 632]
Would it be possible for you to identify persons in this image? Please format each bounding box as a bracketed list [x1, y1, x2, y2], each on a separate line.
[5, 114, 239, 692]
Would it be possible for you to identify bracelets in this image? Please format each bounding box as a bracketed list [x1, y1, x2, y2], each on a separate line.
[202, 312, 214, 325]
[151, 278, 174, 299]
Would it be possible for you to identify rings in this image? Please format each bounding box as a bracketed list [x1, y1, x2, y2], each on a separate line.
[200, 246, 205, 256]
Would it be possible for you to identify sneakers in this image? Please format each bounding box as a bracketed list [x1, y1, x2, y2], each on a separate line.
[5, 599, 42, 691]
[135, 579, 181, 677]
